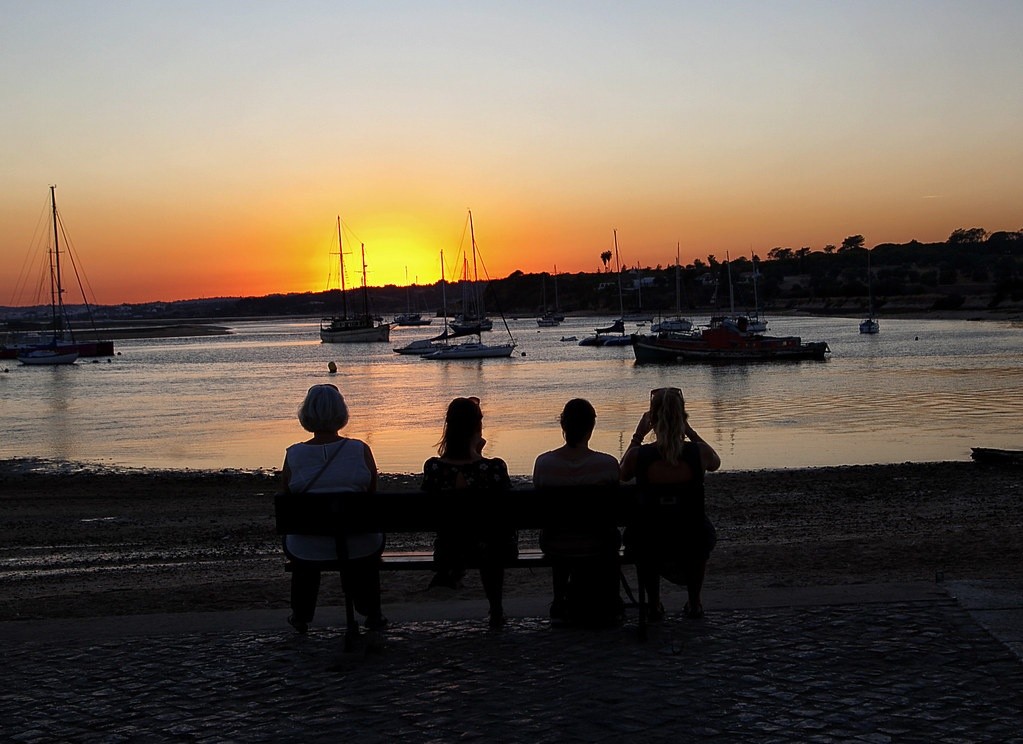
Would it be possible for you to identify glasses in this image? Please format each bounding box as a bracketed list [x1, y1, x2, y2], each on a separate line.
[308, 384, 338, 393]
[651, 388, 684, 411]
[467, 397, 480, 413]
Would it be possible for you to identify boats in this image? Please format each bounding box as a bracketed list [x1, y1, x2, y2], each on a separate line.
[393, 340, 447, 355]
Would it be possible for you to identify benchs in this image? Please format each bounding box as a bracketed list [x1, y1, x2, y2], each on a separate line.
[271, 492, 703, 640]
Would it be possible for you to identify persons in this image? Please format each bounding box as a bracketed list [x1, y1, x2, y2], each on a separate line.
[419, 397, 524, 635]
[533, 398, 622, 628]
[619, 386, 721, 619]
[274, 383, 390, 634]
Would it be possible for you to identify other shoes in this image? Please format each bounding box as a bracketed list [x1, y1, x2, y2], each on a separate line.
[647, 601, 665, 622]
[549, 606, 567, 633]
[684, 600, 703, 618]
[287, 608, 308, 631]
[489, 608, 505, 626]
[364, 614, 388, 627]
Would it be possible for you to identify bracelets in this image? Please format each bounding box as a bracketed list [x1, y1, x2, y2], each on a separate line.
[631, 433, 644, 446]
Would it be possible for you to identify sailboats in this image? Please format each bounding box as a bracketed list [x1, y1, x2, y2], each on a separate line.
[318, 215, 388, 343]
[550, 264, 565, 321]
[355, 240, 494, 332]
[579, 228, 831, 364]
[858, 253, 880, 334]
[419, 209, 517, 358]
[17, 248, 81, 367]
[0, 181, 115, 357]
[535, 276, 560, 326]
